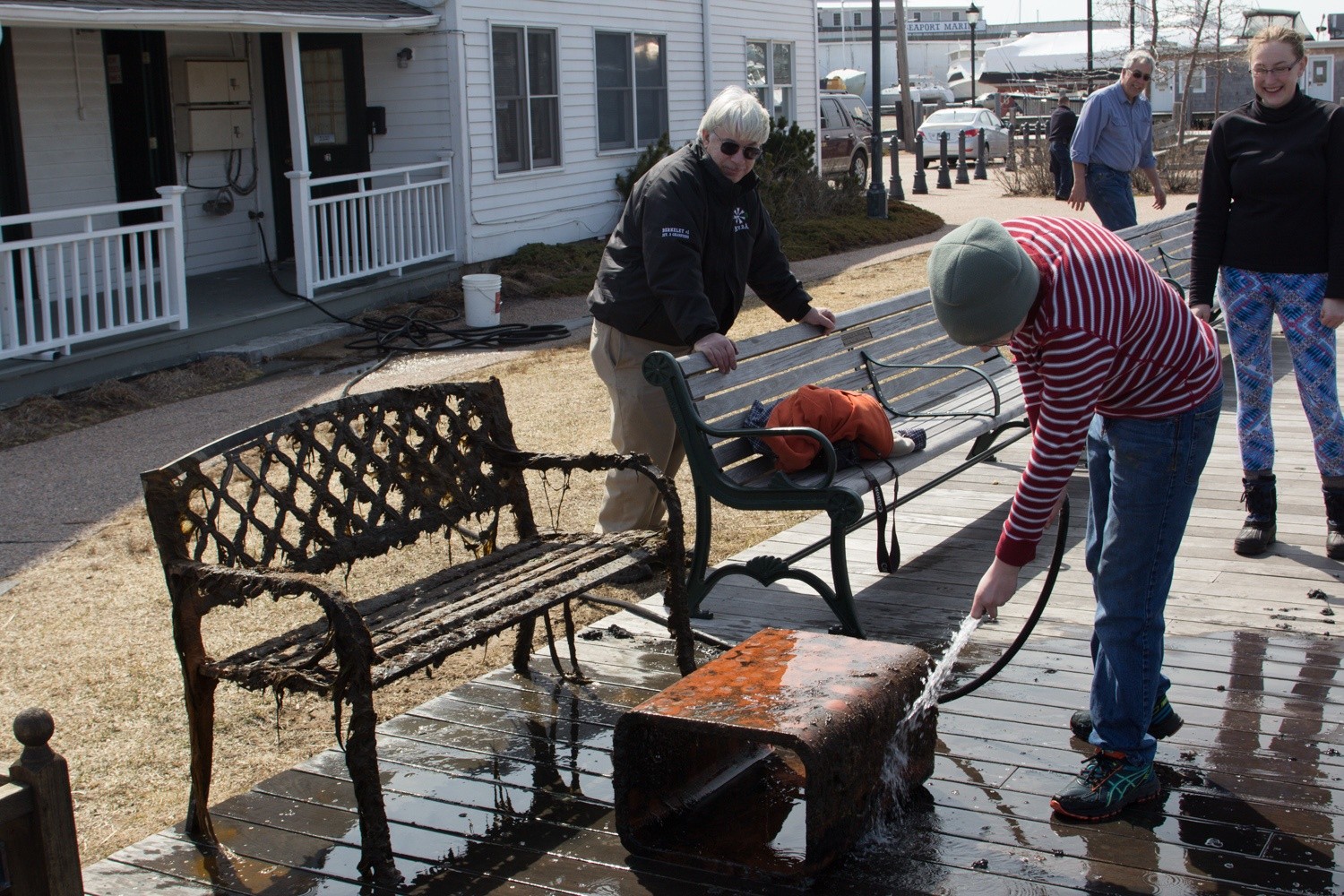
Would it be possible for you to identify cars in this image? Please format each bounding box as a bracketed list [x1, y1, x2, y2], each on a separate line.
[963, 92, 998, 109]
[915, 107, 1009, 169]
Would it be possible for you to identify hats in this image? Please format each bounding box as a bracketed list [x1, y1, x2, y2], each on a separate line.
[926, 217, 1042, 347]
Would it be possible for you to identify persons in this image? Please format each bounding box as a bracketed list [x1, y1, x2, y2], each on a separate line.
[1185, 26, 1344, 556]
[582, 81, 838, 586]
[1067, 48, 1166, 231]
[1048, 95, 1078, 198]
[928, 213, 1225, 823]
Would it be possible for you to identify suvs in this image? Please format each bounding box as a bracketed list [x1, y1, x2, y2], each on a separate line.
[817, 88, 875, 191]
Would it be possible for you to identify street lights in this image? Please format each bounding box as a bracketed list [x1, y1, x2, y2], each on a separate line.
[964, 0, 980, 108]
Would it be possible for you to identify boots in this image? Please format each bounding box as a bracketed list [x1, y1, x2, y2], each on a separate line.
[1234, 474, 1278, 554]
[1322, 484, 1344, 560]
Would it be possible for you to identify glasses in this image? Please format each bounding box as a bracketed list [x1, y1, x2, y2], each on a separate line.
[1127, 69, 1152, 81]
[978, 328, 1015, 347]
[712, 130, 763, 160]
[1249, 58, 1300, 77]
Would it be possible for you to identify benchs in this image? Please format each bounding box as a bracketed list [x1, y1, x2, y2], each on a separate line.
[138, 373, 698, 884]
[642, 203, 1198, 642]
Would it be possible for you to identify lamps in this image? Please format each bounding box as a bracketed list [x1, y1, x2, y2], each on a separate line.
[397, 49, 413, 70]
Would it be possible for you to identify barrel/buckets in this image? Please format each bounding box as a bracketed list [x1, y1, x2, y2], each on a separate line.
[461, 273, 503, 328]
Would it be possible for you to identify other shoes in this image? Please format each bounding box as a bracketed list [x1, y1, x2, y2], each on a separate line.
[576, 552, 653, 586]
[1057, 191, 1070, 199]
[650, 544, 673, 569]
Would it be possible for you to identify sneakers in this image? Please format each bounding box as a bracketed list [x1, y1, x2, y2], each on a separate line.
[1050, 747, 1161, 821]
[1069, 687, 1184, 744]
